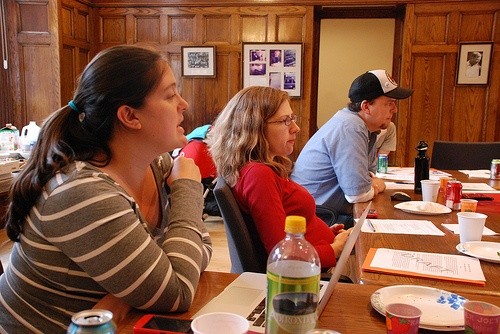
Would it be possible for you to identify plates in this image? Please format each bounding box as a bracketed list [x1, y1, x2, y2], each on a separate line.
[394, 201, 452, 216]
[456, 240, 500, 264]
[370, 285, 471, 331]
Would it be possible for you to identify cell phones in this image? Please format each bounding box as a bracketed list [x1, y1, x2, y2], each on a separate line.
[131, 314, 194, 333]
[361, 207, 379, 218]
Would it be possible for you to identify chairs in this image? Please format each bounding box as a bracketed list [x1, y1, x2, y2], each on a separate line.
[430, 141, 500, 170]
[213, 176, 352, 283]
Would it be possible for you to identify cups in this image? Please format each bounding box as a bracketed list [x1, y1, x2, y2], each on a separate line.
[457, 199, 487, 243]
[385, 303, 422, 334]
[462, 300, 500, 334]
[420, 176, 455, 205]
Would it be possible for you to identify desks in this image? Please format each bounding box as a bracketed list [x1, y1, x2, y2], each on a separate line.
[354, 168, 500, 296]
[90, 271, 500, 334]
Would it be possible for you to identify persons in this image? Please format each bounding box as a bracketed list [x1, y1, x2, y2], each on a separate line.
[466, 52, 482, 78]
[205, 86, 360, 286]
[0, 44, 213, 334]
[290, 70, 415, 231]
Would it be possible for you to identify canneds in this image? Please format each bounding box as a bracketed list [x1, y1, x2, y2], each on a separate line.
[491, 159, 500, 179]
[377, 153, 388, 174]
[67, 309, 118, 334]
[445, 180, 462, 211]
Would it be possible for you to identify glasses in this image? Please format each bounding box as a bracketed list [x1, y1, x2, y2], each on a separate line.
[266, 113, 298, 126]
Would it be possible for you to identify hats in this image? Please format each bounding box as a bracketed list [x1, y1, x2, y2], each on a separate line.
[347, 69, 415, 102]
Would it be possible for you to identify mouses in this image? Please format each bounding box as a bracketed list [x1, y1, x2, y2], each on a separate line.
[390, 192, 411, 201]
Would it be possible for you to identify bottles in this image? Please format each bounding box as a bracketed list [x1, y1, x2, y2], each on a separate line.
[414, 140, 430, 193]
[265, 215, 321, 334]
[21, 121, 42, 158]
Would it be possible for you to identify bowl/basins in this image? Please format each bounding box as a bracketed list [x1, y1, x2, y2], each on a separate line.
[190, 311, 249, 334]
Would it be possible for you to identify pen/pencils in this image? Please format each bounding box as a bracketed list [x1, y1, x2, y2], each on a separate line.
[468, 197, 494, 201]
[395, 182, 414, 184]
[367, 220, 375, 232]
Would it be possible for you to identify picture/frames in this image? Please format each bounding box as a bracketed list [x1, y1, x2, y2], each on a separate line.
[241, 41, 304, 99]
[180, 45, 216, 78]
[455, 41, 493, 87]
